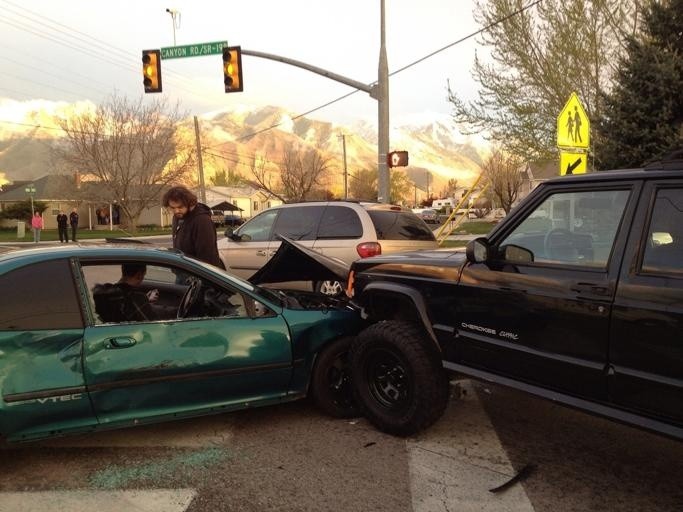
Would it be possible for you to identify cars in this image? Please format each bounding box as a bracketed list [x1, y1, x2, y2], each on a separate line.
[224, 214, 243, 226]
[1, 235, 354, 455]
[420, 207, 441, 225]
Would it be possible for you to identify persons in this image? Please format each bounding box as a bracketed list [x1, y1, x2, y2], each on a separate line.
[93, 261, 160, 322]
[162, 187, 236, 295]
[96, 204, 120, 225]
[32, 210, 43, 243]
[70, 208, 79, 242]
[57, 209, 68, 243]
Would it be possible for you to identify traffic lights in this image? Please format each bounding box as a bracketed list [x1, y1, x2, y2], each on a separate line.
[220, 44, 245, 93]
[387, 150, 409, 168]
[140, 47, 161, 94]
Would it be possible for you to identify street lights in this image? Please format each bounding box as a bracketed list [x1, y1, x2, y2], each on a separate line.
[24, 181, 36, 217]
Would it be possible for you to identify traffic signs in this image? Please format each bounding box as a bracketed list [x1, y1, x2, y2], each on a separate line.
[161, 38, 228, 61]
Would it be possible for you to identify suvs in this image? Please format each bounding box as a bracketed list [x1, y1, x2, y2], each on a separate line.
[338, 161, 681, 443]
[213, 198, 442, 286]
